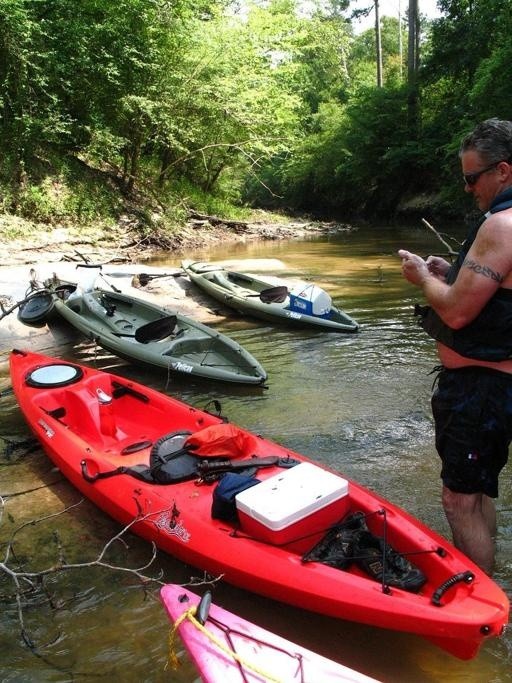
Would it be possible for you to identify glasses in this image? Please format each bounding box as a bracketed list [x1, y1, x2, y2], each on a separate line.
[463, 162, 500, 186]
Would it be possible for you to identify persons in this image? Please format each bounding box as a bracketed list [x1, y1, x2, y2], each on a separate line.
[398, 118, 512, 578]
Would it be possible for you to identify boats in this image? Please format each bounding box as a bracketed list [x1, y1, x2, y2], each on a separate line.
[27, 268, 271, 392]
[158, 581, 384, 683]
[7, 345, 511, 662]
[180, 257, 360, 334]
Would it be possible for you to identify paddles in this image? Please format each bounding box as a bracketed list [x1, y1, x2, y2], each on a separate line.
[139, 272, 184, 285]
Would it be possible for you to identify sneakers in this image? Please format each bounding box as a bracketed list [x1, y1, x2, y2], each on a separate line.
[305, 511, 369, 570]
[351, 531, 426, 588]
